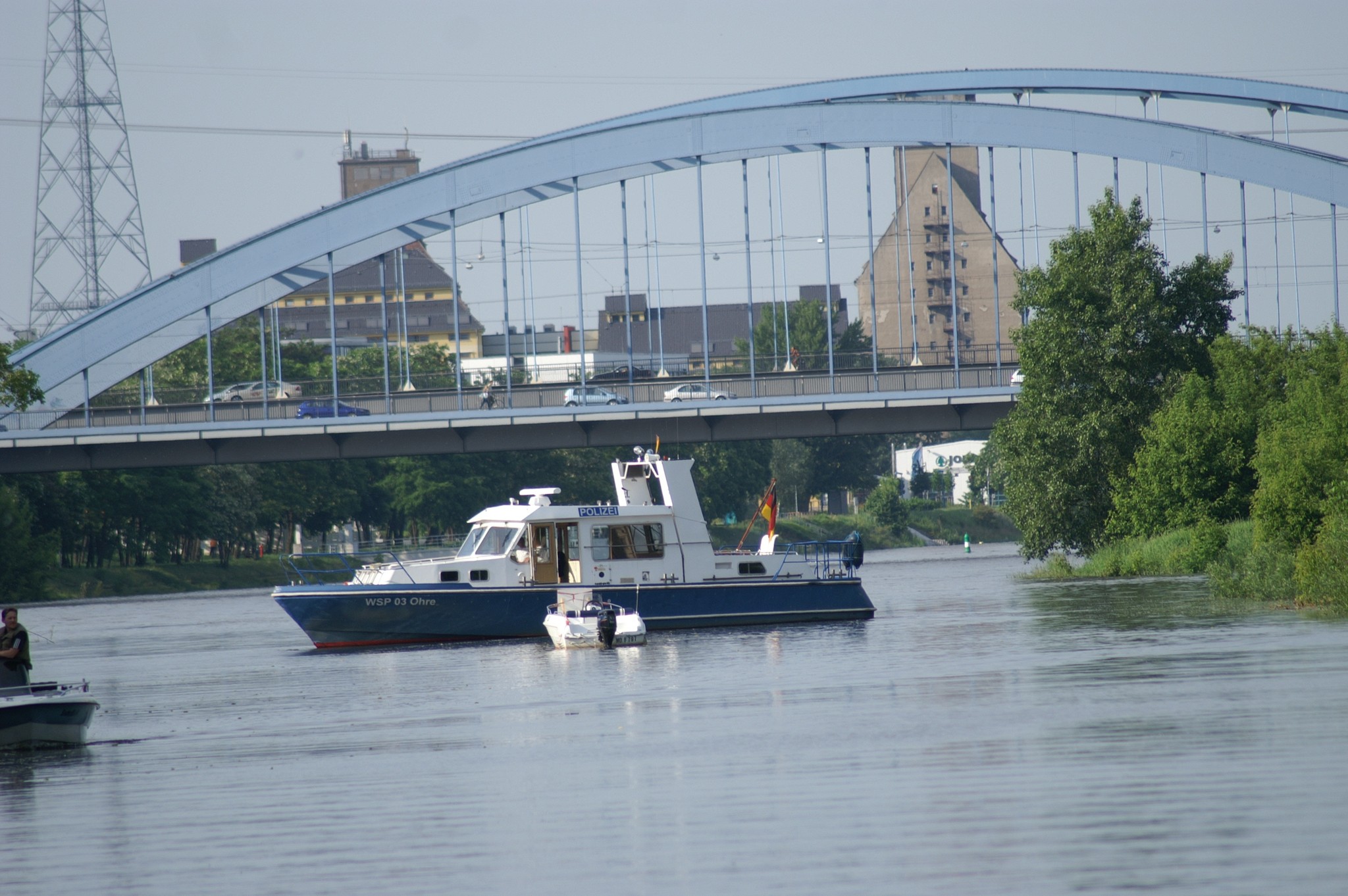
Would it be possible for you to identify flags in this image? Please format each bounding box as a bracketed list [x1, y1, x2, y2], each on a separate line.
[760, 481, 779, 540]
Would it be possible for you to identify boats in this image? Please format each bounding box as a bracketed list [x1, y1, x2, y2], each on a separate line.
[267, 452, 876, 653]
[0, 676, 99, 746]
[544, 593, 650, 651]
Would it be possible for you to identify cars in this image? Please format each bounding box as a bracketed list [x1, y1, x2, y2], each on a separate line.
[296, 396, 370, 420]
[204, 381, 306, 404]
[662, 383, 737, 402]
[565, 386, 631, 408]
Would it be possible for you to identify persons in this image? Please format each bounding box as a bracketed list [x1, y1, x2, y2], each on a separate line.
[0, 607, 31, 684]
[478, 379, 495, 411]
[533, 538, 550, 563]
[515, 536, 531, 563]
